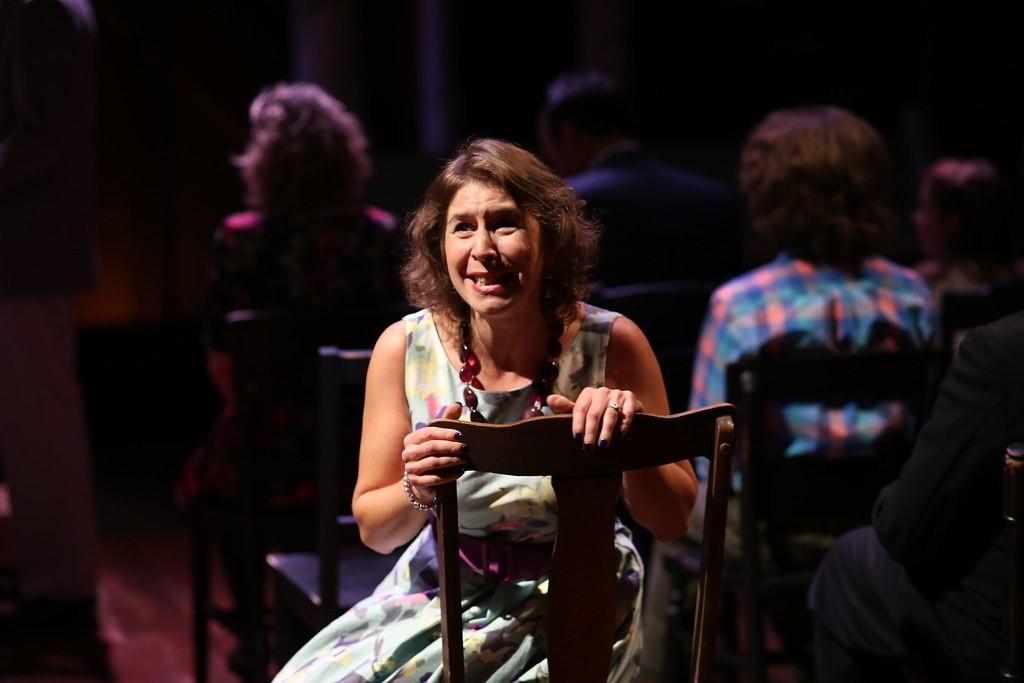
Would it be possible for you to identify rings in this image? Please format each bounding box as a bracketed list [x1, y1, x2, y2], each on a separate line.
[606, 403, 621, 414]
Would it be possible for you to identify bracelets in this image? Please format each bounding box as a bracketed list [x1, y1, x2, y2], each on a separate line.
[403, 468, 439, 510]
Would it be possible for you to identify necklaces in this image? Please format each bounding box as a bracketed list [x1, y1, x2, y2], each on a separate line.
[458, 306, 566, 425]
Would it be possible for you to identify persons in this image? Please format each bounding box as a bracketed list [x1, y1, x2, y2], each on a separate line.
[807, 312, 1023, 683]
[632, 102, 941, 681]
[183, 79, 403, 669]
[537, 66, 751, 292]
[270, 135, 698, 681]
[901, 158, 1022, 376]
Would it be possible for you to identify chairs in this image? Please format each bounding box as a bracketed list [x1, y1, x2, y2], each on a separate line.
[431, 394, 737, 683]
[188, 305, 376, 683]
